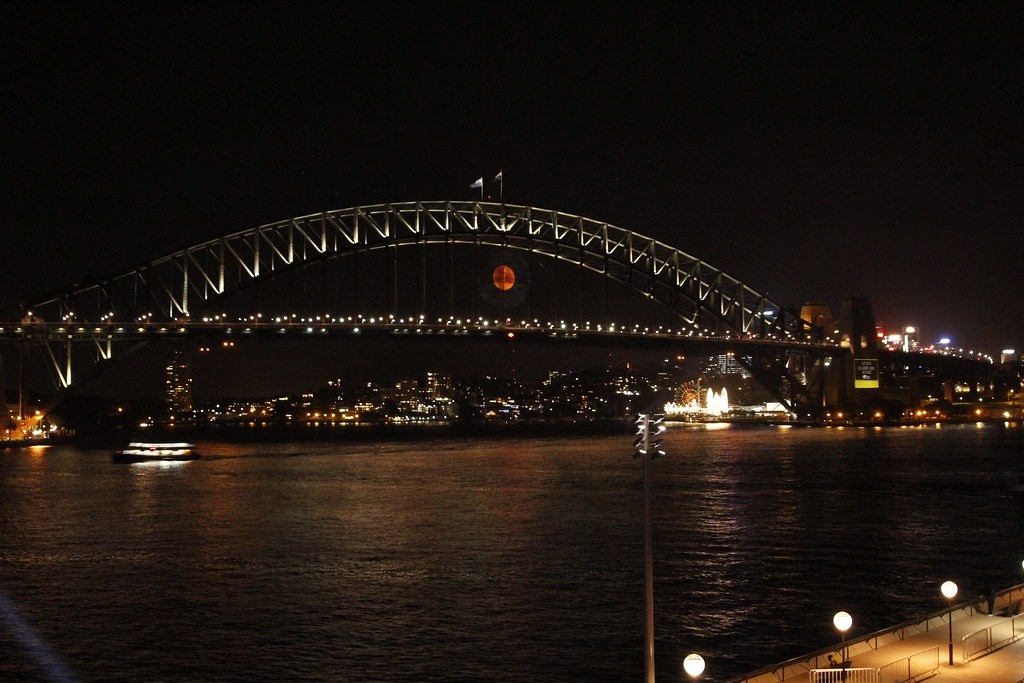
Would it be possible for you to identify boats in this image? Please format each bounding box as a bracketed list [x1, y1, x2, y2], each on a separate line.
[113, 440, 199, 465]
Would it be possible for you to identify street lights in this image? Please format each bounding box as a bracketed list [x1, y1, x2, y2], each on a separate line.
[633, 412, 667, 683]
[833, 611, 853, 683]
[941, 581, 959, 665]
